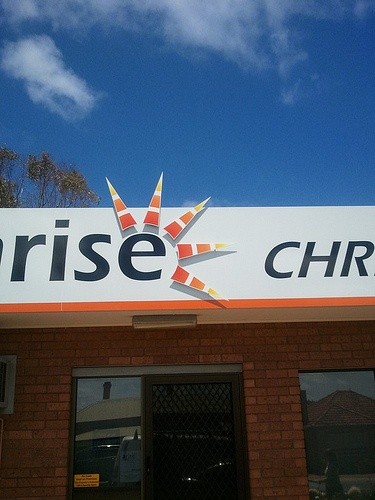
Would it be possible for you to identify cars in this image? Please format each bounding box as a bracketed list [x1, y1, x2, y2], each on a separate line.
[74, 445, 120, 483]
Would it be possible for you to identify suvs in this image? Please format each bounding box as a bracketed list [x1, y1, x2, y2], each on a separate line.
[113, 434, 143, 486]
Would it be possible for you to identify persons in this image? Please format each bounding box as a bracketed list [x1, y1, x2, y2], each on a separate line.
[324, 448, 344, 499]
